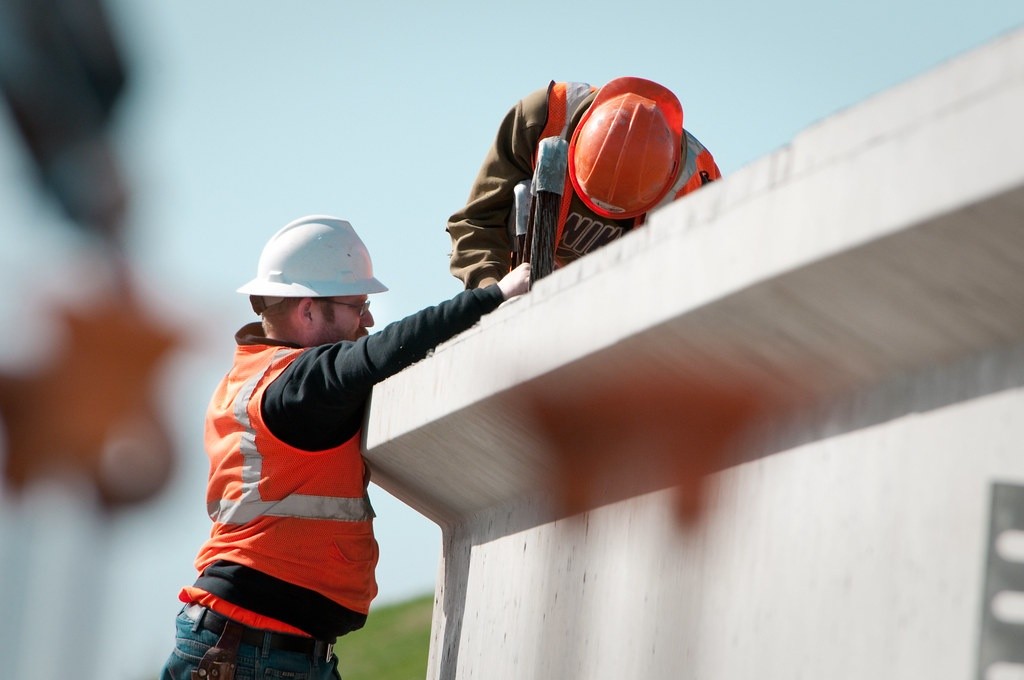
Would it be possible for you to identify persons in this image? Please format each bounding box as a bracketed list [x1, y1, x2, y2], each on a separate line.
[159, 215, 531, 680]
[0, 1, 187, 504]
[445, 76, 721, 290]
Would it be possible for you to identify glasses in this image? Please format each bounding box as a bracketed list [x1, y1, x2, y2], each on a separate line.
[317, 297, 370, 317]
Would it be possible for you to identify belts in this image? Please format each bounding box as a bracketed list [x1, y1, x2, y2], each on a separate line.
[183, 600, 334, 664]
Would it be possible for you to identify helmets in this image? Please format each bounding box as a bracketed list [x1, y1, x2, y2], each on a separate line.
[569, 76, 685, 218]
[237, 215, 388, 298]
